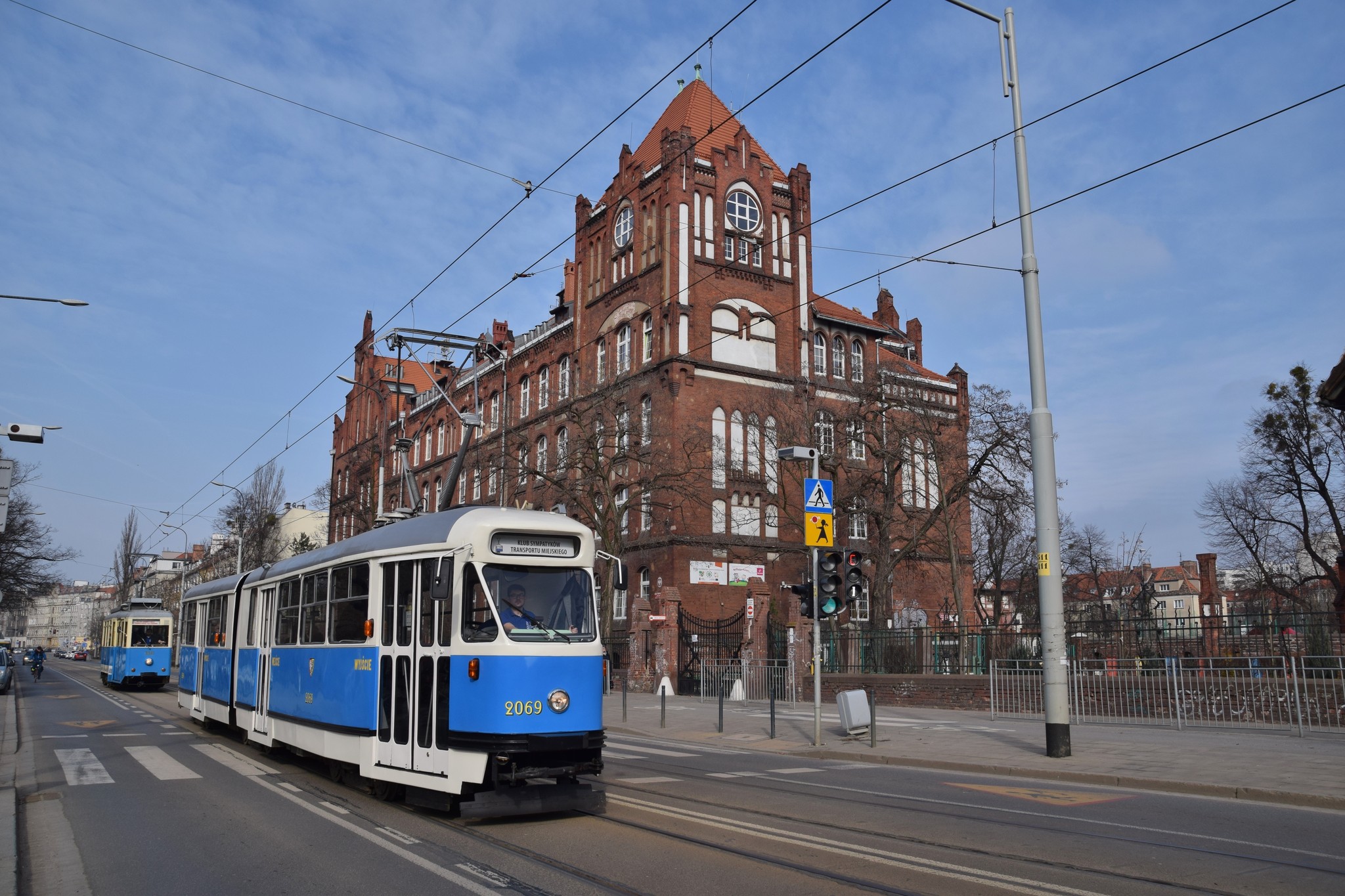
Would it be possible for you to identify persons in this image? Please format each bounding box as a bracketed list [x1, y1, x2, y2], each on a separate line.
[29, 646, 47, 679]
[210, 622, 221, 646]
[136, 628, 165, 647]
[498, 584, 578, 633]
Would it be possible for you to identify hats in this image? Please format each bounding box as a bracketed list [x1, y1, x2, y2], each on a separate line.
[38, 646, 42, 650]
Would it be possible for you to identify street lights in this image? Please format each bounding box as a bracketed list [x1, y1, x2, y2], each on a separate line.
[61, 480, 246, 659]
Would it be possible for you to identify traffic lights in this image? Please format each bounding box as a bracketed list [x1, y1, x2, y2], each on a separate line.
[817, 549, 843, 617]
[843, 547, 863, 601]
[792, 582, 817, 620]
[53, 629, 57, 634]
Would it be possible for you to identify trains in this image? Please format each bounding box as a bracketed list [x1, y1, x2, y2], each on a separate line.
[100, 327, 632, 824]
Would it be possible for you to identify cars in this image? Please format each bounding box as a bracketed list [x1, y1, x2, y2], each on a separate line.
[0, 645, 87, 695]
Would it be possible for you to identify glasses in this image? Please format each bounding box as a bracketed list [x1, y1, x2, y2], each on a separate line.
[509, 594, 525, 599]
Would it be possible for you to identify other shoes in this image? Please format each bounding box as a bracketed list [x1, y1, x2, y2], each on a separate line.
[38, 675, 41, 679]
[32, 670, 35, 675]
